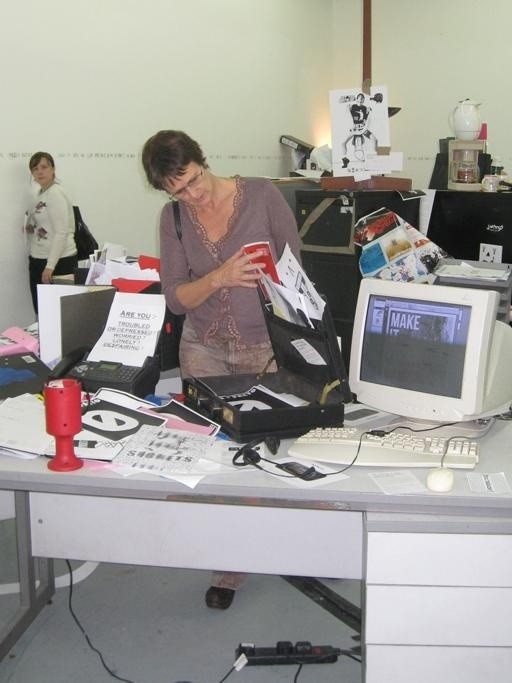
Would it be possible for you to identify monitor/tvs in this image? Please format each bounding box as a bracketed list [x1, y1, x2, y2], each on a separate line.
[349, 278, 512, 439]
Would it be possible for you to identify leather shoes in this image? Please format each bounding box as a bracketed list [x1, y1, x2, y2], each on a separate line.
[205, 585, 236, 609]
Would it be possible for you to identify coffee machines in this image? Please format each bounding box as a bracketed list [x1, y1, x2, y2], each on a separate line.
[448, 139, 486, 192]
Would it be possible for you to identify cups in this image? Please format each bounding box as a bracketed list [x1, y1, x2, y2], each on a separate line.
[481, 175, 497, 192]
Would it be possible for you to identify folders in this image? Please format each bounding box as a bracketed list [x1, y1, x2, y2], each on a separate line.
[280, 135, 315, 154]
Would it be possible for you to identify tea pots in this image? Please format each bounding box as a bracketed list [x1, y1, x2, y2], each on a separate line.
[448, 101, 481, 138]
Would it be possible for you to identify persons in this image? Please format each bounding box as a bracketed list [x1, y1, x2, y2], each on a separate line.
[341, 93, 378, 168]
[25, 152, 81, 320]
[141, 129, 302, 609]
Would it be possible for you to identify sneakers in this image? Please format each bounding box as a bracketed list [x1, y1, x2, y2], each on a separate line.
[342, 157, 349, 168]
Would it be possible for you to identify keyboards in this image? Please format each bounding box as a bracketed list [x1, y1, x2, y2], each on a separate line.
[288, 427, 479, 468]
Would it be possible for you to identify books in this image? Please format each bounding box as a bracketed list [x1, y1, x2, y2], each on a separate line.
[240, 241, 282, 301]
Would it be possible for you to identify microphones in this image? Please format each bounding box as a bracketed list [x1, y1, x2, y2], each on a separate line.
[253, 463, 315, 478]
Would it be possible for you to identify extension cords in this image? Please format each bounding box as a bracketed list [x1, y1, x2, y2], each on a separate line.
[235, 645, 338, 666]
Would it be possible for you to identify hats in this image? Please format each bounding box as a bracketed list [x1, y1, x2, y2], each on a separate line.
[370, 93, 383, 103]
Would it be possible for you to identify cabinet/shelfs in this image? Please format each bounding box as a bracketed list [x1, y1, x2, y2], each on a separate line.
[297, 187, 512, 325]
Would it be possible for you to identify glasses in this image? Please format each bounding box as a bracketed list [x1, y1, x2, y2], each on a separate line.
[169, 165, 204, 202]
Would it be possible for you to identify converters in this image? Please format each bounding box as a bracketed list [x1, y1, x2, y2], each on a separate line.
[296, 641, 311, 654]
[239, 642, 256, 657]
[277, 641, 293, 655]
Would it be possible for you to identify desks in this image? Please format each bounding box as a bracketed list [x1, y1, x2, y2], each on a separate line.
[0, 365, 511, 683]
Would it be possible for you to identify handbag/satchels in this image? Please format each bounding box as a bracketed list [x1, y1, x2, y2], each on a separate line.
[72, 206, 98, 261]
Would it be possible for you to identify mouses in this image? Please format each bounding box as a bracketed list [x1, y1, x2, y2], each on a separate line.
[426, 467, 454, 492]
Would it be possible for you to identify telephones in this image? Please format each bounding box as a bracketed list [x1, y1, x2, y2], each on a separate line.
[47, 345, 161, 399]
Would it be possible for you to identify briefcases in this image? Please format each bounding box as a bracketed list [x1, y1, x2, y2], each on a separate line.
[182, 277, 352, 444]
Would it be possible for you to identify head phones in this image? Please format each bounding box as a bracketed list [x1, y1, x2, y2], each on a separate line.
[233, 436, 280, 466]
